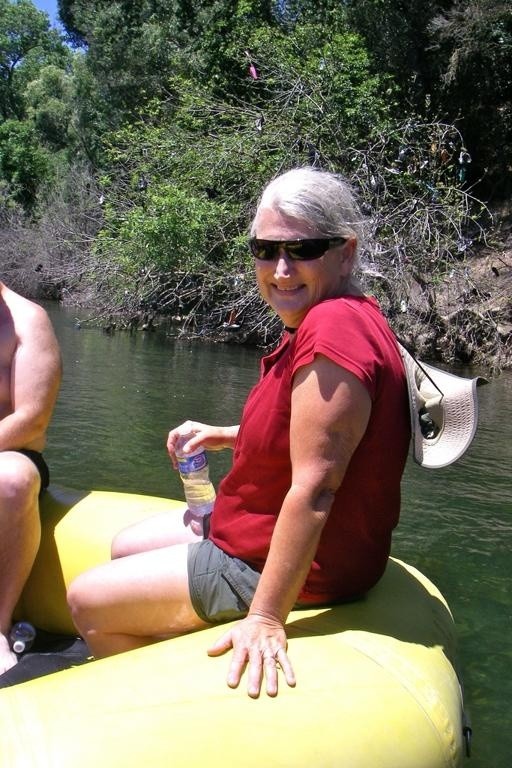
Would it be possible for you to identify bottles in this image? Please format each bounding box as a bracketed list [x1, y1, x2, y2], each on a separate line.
[174, 422, 219, 516]
[7, 622, 36, 655]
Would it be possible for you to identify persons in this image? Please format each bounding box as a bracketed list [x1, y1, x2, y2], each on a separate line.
[1, 279, 62, 674]
[63, 160, 415, 701]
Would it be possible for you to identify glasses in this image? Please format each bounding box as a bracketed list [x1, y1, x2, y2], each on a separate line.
[248, 236, 347, 260]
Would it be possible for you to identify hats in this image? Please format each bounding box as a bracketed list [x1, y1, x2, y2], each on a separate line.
[396, 340, 489, 470]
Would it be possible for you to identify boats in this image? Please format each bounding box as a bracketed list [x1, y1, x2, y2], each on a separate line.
[0, 480, 470, 768]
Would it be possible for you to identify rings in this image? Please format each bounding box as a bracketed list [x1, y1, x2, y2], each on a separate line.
[262, 655, 276, 660]
